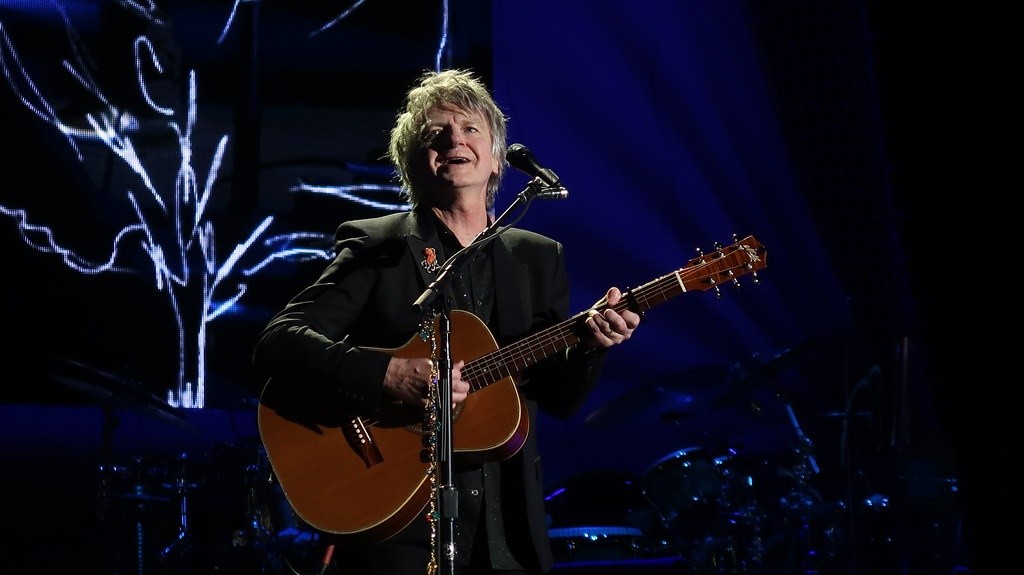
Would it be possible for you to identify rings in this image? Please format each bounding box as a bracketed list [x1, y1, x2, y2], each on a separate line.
[606, 329, 613, 335]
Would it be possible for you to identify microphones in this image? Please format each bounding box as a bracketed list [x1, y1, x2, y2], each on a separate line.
[505, 143, 569, 198]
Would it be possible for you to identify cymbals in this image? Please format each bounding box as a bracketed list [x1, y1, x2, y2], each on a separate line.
[586, 363, 739, 432]
[706, 327, 843, 415]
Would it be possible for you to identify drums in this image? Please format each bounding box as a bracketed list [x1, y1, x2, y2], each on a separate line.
[711, 506, 809, 575]
[642, 439, 748, 538]
[544, 469, 649, 559]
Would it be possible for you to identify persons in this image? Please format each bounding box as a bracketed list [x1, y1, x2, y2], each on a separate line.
[254, 68, 641, 575]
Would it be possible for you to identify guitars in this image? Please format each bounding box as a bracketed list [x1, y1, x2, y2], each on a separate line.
[255, 226, 777, 548]
